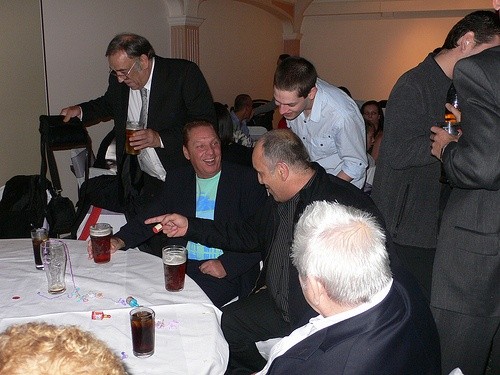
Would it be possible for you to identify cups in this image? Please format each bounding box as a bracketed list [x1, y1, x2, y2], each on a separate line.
[129, 307, 155, 358]
[31, 228, 50, 270]
[162, 245, 187, 292]
[435, 121, 460, 137]
[90, 223, 111, 264]
[40, 240, 67, 294]
[126, 120, 144, 155]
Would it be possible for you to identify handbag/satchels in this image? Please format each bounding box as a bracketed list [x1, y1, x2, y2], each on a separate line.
[0, 175, 49, 239]
[39, 115, 92, 151]
[71, 174, 124, 233]
[46, 188, 77, 237]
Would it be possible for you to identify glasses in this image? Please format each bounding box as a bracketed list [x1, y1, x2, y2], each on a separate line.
[109, 60, 137, 80]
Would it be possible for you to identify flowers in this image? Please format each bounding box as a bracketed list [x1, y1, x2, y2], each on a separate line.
[232, 129, 254, 147]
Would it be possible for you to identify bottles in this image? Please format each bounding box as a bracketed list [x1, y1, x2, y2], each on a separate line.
[445, 82, 461, 124]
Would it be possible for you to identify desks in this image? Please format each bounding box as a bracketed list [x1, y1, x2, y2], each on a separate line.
[1, 237, 229, 375]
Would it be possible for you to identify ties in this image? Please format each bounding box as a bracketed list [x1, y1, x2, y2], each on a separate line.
[136, 88, 147, 130]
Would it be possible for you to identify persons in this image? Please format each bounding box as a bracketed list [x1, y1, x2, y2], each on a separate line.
[338, 86, 387, 191]
[215, 54, 291, 166]
[228, 200, 441, 375]
[60, 32, 214, 254]
[86, 121, 263, 308]
[144, 128, 388, 375]
[0, 322, 126, 375]
[369, 7, 500, 375]
[272, 56, 368, 190]
